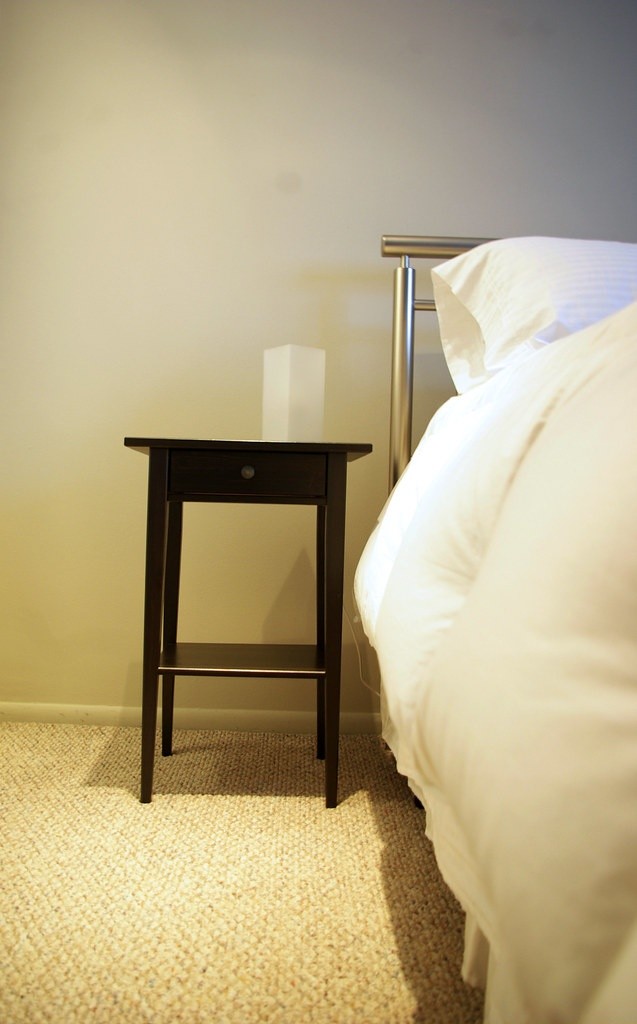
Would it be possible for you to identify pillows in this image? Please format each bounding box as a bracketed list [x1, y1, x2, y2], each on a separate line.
[429, 235, 637, 396]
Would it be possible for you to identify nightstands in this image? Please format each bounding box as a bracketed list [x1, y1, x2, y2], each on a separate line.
[123, 436, 373, 809]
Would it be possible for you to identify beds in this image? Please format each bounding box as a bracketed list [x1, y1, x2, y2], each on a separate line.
[352, 235, 637, 1024]
